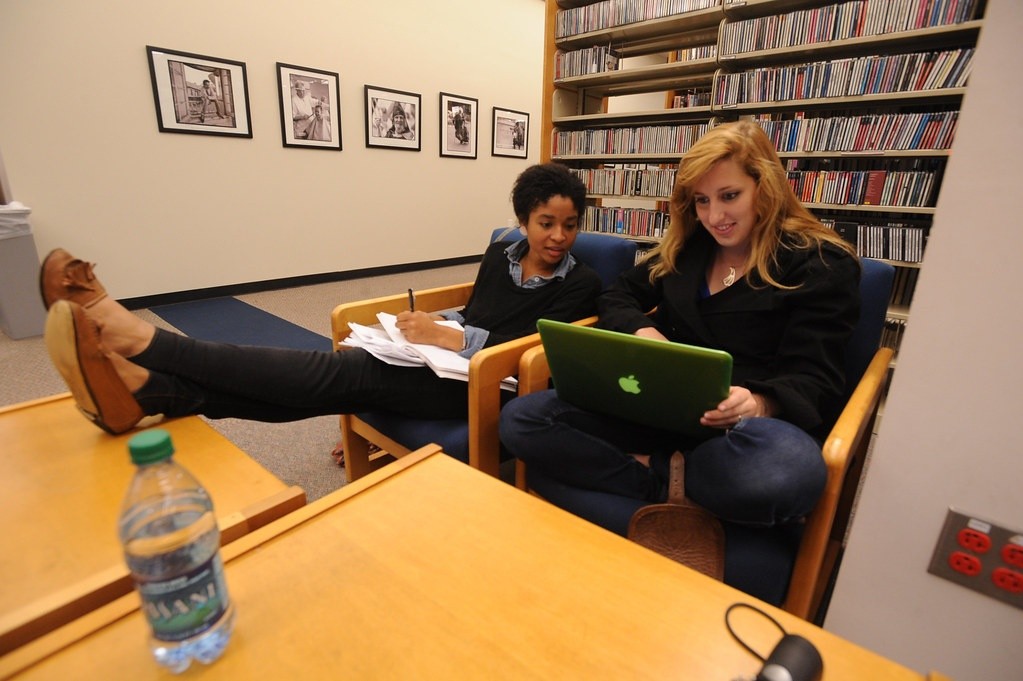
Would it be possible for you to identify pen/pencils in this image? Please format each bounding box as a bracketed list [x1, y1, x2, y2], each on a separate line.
[408, 289, 414, 312]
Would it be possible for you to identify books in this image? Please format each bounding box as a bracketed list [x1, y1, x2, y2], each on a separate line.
[581, 206, 673, 239]
[892, 269, 919, 312]
[560, 0, 984, 78]
[542, 158, 940, 208]
[634, 248, 656, 267]
[563, 49, 980, 105]
[554, 110, 963, 149]
[556, 0, 731, 39]
[881, 322, 903, 361]
[814, 214, 923, 266]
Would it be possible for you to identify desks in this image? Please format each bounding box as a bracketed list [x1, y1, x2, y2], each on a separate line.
[0, 392, 311, 647]
[0, 443, 954, 681]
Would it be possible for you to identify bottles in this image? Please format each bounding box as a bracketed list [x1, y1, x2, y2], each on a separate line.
[116, 427, 237, 668]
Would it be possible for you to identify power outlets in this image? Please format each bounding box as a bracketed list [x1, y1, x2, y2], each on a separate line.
[927, 508, 1023, 617]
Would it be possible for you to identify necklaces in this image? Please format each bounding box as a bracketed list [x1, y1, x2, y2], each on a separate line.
[716, 252, 757, 287]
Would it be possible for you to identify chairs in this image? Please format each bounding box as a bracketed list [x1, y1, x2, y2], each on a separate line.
[517, 254, 900, 618]
[330, 223, 657, 491]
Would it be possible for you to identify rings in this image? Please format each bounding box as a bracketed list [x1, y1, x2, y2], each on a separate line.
[738, 415, 742, 423]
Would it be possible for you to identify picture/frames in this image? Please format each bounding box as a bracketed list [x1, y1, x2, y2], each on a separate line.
[363, 82, 423, 153]
[145, 44, 255, 140]
[438, 90, 479, 161]
[276, 60, 343, 152]
[491, 105, 530, 159]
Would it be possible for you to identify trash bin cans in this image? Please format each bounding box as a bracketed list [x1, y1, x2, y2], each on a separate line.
[0, 206, 53, 339]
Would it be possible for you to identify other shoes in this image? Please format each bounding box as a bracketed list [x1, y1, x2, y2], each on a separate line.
[44, 300, 145, 435]
[39, 248, 109, 312]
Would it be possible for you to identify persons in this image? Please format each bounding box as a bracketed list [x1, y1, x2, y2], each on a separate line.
[510, 122, 523, 150]
[452, 108, 467, 143]
[304, 106, 332, 140]
[37, 162, 602, 446]
[200, 80, 225, 123]
[386, 101, 415, 140]
[291, 80, 326, 139]
[499, 121, 861, 604]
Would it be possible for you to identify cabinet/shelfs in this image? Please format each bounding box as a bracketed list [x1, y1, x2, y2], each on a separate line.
[664, 45, 716, 109]
[540, 0, 988, 370]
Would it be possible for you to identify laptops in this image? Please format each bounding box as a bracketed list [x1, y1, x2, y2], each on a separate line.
[536, 317, 733, 436]
